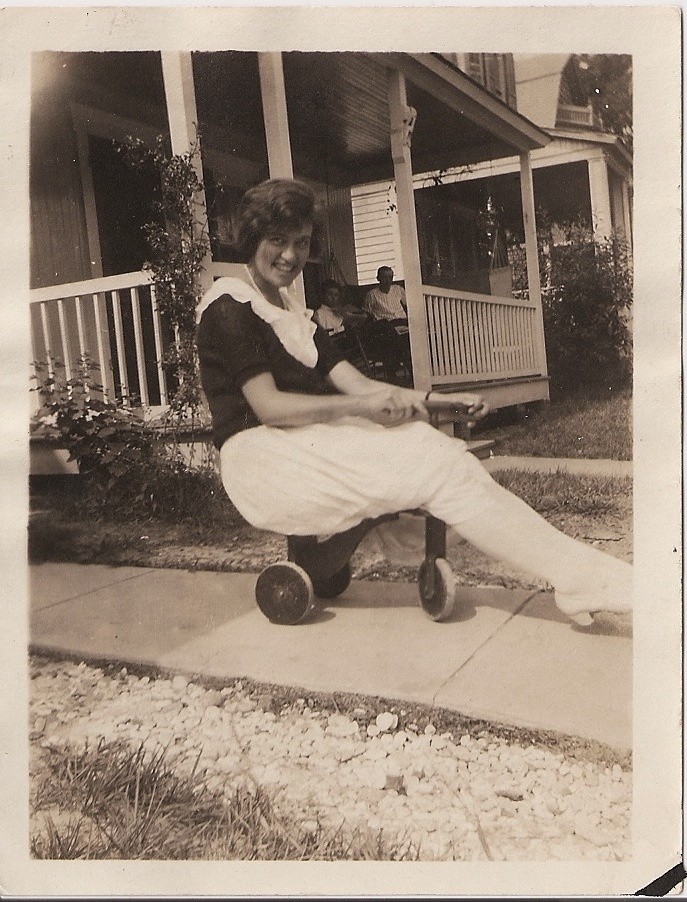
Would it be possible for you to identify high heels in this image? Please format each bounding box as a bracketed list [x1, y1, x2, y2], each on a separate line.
[554, 565, 633, 626]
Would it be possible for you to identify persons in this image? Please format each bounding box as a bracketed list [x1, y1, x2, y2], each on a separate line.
[313, 266, 413, 378]
[194, 177, 633, 627]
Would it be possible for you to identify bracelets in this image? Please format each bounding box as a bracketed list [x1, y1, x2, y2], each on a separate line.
[425, 391, 431, 400]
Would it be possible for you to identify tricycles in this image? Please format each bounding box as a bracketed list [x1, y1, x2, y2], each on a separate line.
[251, 395, 483, 627]
